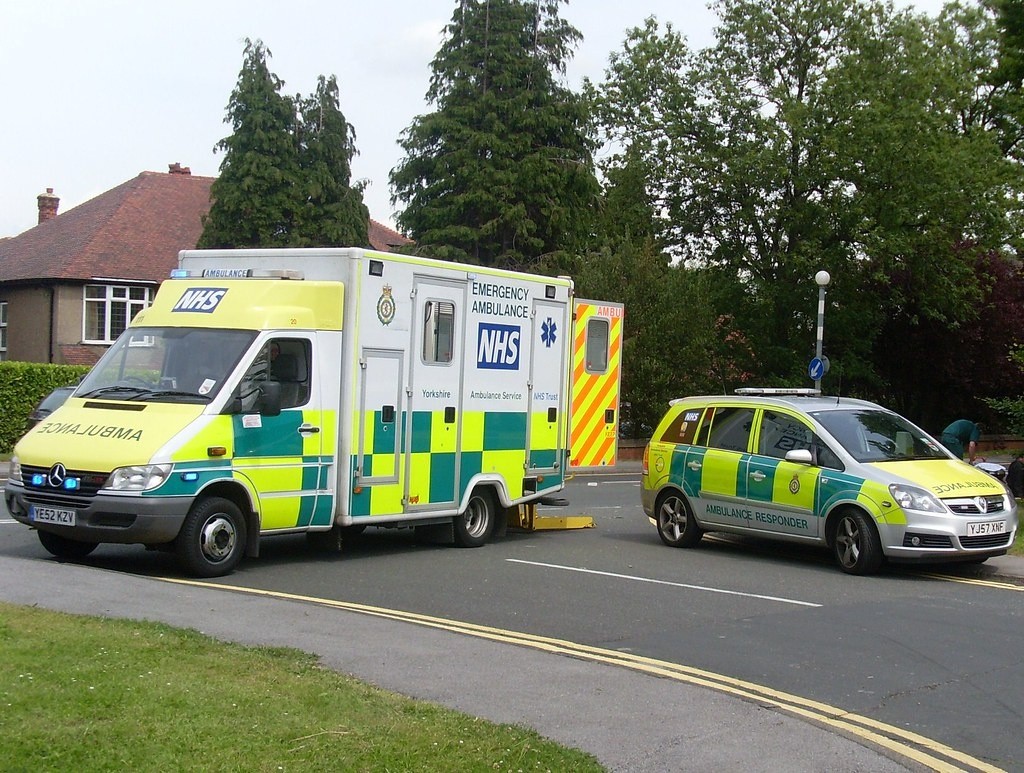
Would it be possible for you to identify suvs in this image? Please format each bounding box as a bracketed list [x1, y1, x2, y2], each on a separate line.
[28, 386, 77, 432]
[640, 385, 1019, 575]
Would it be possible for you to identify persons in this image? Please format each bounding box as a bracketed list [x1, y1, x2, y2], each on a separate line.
[1007, 453, 1024, 499]
[942, 419, 985, 465]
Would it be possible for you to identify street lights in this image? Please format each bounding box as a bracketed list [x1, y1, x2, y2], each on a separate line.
[814, 269, 831, 401]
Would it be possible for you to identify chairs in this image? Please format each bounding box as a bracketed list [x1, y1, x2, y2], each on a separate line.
[268, 354, 306, 411]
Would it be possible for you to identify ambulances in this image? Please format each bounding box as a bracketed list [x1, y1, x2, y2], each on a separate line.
[5, 246, 625, 573]
